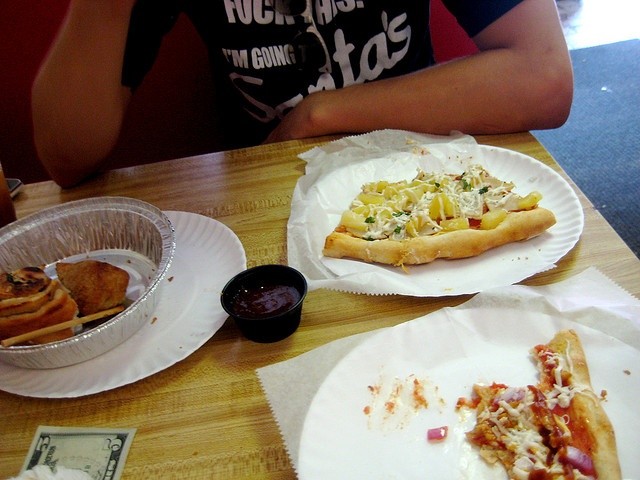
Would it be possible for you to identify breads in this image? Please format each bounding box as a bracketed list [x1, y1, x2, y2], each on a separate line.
[56, 259, 130, 316]
[0, 266, 84, 345]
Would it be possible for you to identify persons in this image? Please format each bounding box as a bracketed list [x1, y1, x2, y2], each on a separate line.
[29, 1, 572, 188]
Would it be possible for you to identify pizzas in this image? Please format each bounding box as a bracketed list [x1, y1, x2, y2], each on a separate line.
[464, 329, 622, 480]
[322, 164, 556, 265]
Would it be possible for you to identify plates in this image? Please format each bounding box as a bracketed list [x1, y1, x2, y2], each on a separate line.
[307, 144, 583, 299]
[0, 209, 248, 398]
[297, 306, 640, 480]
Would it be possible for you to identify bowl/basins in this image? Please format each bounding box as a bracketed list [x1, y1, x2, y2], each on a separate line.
[220, 263, 307, 344]
[1, 194, 177, 367]
